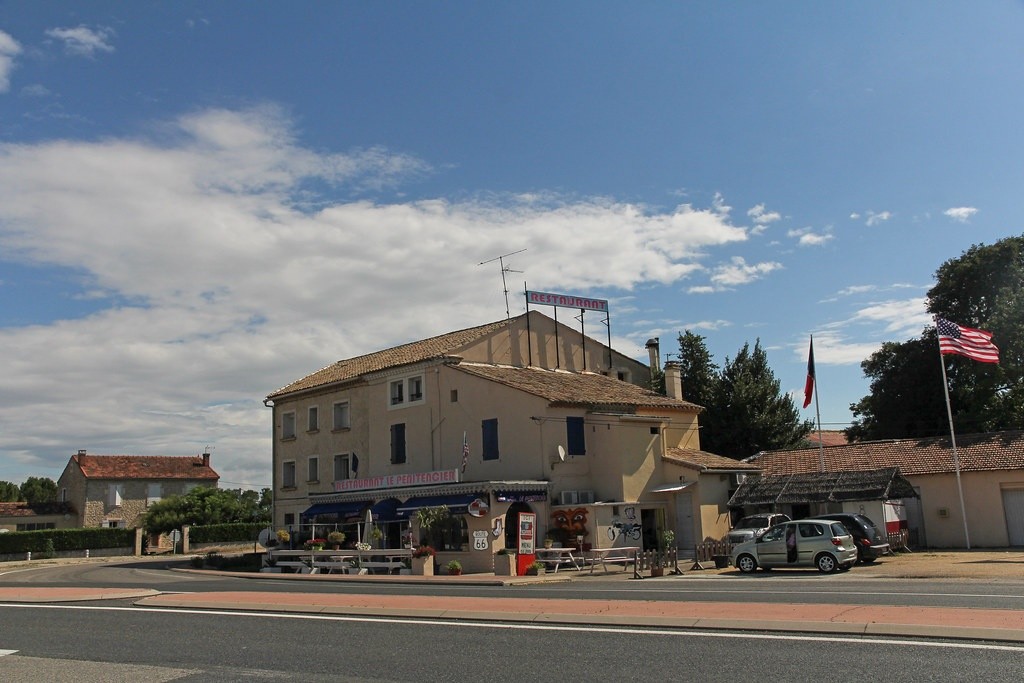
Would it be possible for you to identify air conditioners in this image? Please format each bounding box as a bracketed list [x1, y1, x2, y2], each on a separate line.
[559, 491, 578, 505]
[578, 489, 595, 504]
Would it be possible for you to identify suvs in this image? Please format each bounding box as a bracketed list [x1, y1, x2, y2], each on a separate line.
[723, 513, 792, 546]
[730, 520, 859, 575]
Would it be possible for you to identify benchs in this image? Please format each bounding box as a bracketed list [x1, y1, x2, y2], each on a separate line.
[533, 558, 571, 574]
[586, 556, 628, 574]
[543, 557, 585, 571]
[598, 557, 642, 575]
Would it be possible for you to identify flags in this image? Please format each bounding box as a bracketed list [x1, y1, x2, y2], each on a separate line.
[934, 314, 1000, 364]
[802, 335, 814, 408]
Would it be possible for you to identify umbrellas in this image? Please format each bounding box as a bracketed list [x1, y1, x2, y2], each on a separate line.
[361, 508, 376, 547]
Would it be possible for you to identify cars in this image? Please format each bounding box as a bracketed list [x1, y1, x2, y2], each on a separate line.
[808, 513, 890, 564]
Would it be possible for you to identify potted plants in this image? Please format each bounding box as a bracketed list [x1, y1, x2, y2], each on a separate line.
[260, 528, 553, 575]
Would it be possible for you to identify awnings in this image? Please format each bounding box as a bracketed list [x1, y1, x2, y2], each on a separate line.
[300, 500, 376, 517]
[396, 492, 487, 511]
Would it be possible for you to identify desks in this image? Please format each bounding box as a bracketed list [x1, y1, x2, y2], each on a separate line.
[589, 547, 641, 576]
[534, 547, 581, 573]
